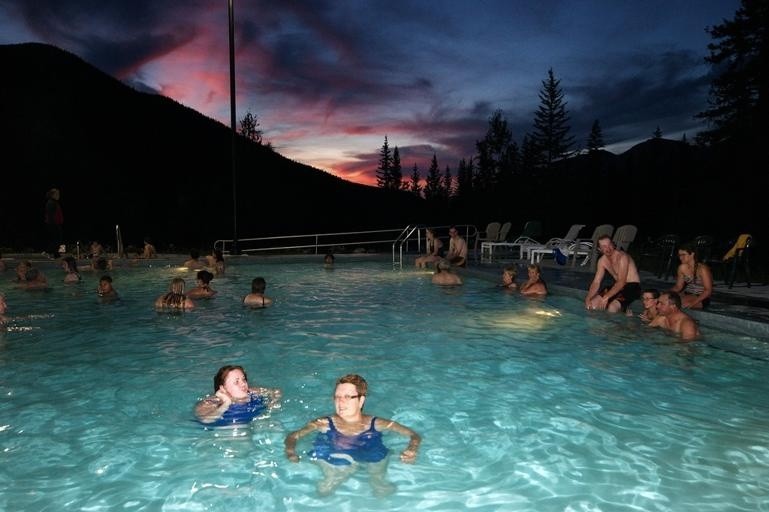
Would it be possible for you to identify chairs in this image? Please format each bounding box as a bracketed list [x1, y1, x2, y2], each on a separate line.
[663, 233, 711, 280]
[520, 227, 587, 260]
[531, 224, 614, 264]
[474, 222, 501, 256]
[571, 225, 638, 264]
[702, 234, 752, 289]
[481, 221, 539, 262]
[492, 222, 513, 257]
[637, 234, 680, 278]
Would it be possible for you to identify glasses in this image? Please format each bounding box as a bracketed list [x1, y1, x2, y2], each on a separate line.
[335, 393, 361, 402]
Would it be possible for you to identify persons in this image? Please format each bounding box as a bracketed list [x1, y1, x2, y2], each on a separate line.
[669, 243, 712, 311]
[11, 240, 117, 298]
[520, 264, 548, 296]
[212, 365, 248, 402]
[626, 289, 661, 325]
[415, 226, 467, 285]
[584, 236, 641, 313]
[156, 248, 225, 309]
[142, 239, 157, 259]
[496, 266, 518, 289]
[650, 292, 702, 341]
[324, 254, 335, 264]
[335, 374, 367, 421]
[243, 277, 272, 305]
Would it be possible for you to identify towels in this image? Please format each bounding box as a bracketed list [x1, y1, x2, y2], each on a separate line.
[723, 234, 752, 261]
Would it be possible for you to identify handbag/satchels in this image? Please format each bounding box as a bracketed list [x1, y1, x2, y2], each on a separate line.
[552, 245, 569, 266]
